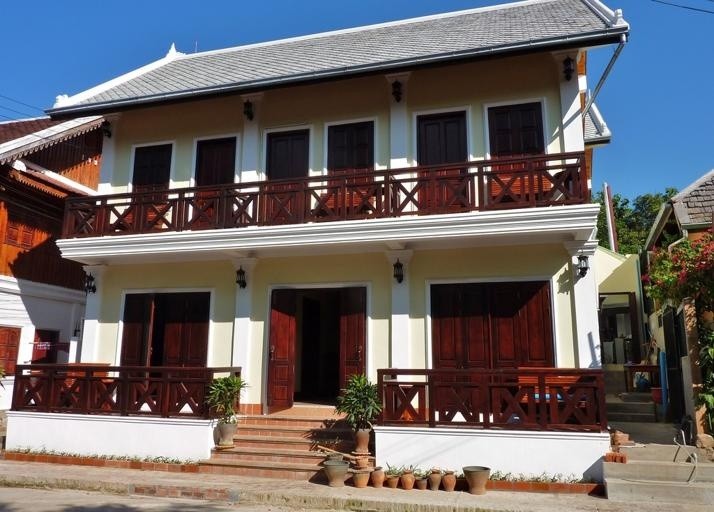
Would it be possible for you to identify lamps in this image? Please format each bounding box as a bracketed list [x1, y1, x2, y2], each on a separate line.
[392, 80, 402, 102]
[393, 257, 403, 282]
[243, 99, 254, 119]
[101, 117, 113, 137]
[577, 250, 590, 277]
[85, 273, 96, 294]
[563, 55, 575, 81]
[236, 265, 246, 288]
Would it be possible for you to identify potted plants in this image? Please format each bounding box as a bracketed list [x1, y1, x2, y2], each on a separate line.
[206, 376, 252, 447]
[335, 373, 384, 453]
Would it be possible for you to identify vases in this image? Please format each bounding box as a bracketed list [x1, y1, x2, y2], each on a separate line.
[323, 453, 490, 495]
[650, 386, 668, 405]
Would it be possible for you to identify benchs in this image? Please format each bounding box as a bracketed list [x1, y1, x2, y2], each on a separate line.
[58, 364, 109, 392]
[519, 367, 604, 423]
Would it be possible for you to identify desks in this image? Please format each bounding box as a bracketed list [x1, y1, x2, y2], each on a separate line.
[624, 365, 659, 394]
